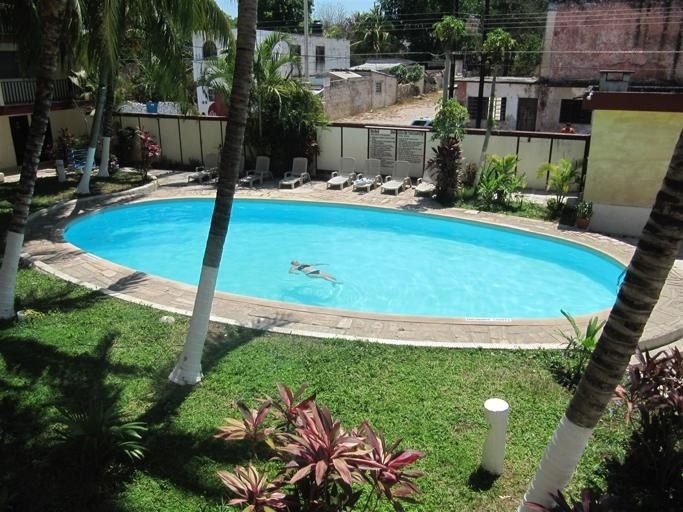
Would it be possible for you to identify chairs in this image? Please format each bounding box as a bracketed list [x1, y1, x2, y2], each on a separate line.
[184, 152, 441, 199]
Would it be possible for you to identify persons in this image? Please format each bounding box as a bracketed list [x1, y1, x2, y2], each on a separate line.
[560, 122, 574, 133]
[289, 259, 338, 285]
